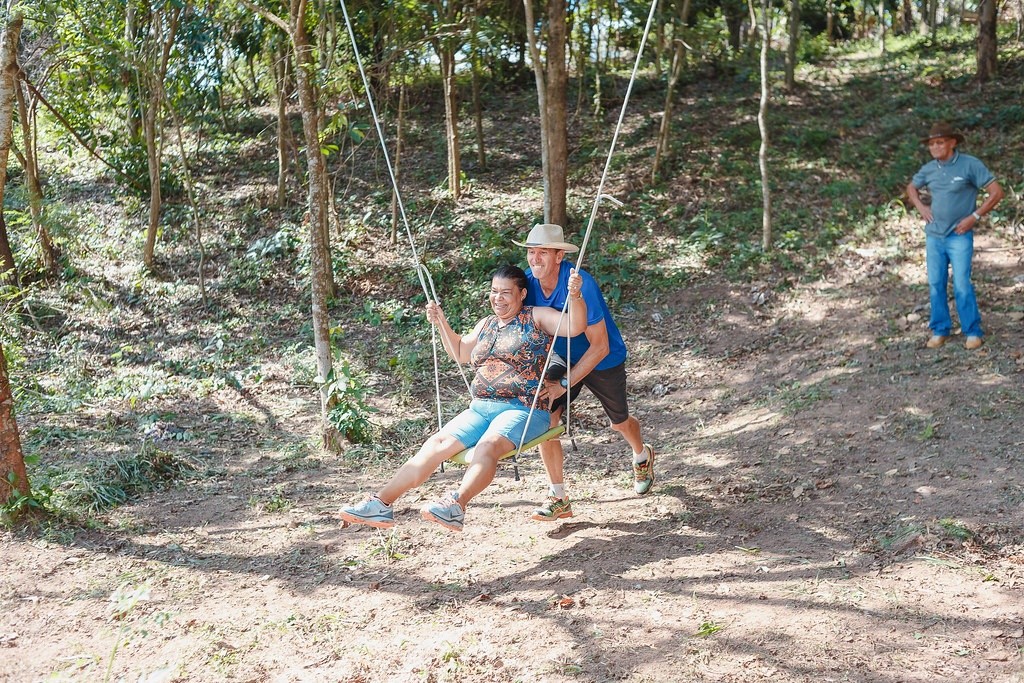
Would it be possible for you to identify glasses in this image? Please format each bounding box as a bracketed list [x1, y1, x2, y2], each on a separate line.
[928, 138, 952, 146]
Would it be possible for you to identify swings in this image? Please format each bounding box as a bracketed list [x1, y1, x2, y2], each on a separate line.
[332, 0, 676, 468]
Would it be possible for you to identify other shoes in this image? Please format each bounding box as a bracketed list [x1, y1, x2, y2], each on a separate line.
[966, 337, 982, 349]
[927, 336, 951, 349]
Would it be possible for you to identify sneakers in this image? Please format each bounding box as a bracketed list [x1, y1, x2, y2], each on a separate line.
[531, 496, 573, 521]
[632, 444, 654, 497]
[339, 492, 394, 528]
[421, 493, 466, 531]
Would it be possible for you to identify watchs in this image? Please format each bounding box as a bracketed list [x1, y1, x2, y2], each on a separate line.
[559, 376, 571, 391]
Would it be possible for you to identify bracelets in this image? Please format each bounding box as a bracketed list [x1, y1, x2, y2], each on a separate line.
[973, 212, 980, 220]
[568, 292, 583, 304]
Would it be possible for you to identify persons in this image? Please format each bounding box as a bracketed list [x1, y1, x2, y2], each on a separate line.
[907, 121, 1003, 350]
[338, 265, 587, 532]
[510, 223, 654, 521]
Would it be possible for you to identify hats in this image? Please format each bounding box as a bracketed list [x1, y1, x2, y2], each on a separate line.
[921, 123, 964, 144]
[511, 223, 579, 253]
